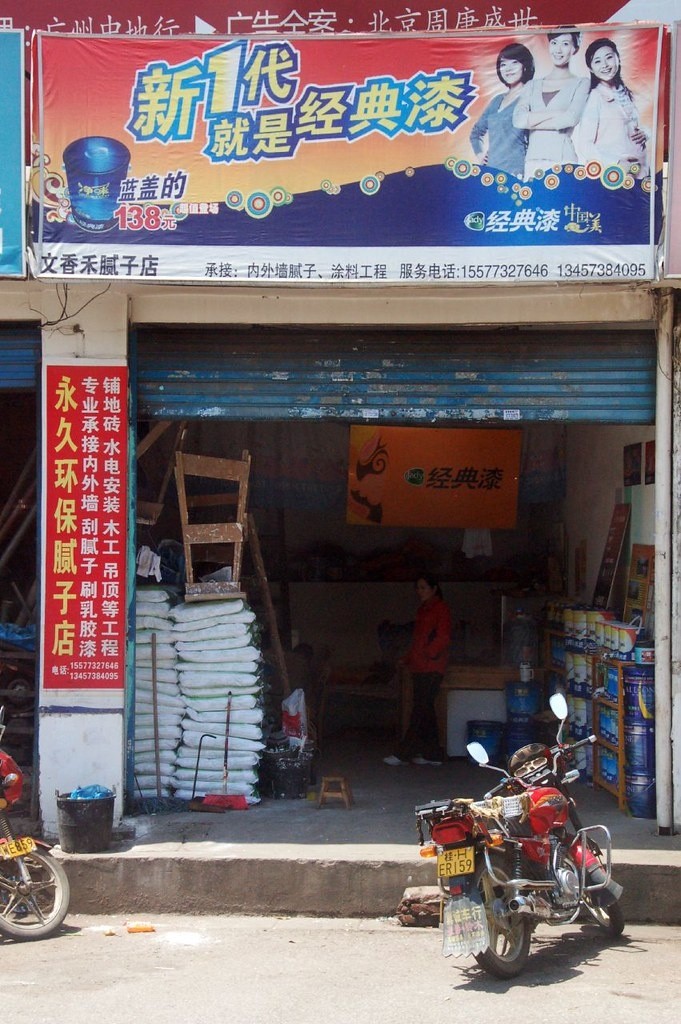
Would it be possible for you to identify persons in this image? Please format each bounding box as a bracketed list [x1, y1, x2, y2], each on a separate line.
[383, 576, 450, 767]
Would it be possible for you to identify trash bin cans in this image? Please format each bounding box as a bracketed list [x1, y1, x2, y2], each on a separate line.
[54, 785, 117, 853]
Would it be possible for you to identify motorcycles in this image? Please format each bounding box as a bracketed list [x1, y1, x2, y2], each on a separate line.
[412, 692, 626, 981]
[0, 704, 73, 940]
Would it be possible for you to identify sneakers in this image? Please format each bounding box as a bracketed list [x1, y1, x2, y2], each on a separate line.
[383, 755, 410, 767]
[411, 757, 442, 766]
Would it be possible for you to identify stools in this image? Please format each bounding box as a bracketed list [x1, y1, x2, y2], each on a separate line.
[317, 773, 354, 810]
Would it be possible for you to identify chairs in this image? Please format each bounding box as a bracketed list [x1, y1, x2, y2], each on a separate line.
[313, 661, 333, 757]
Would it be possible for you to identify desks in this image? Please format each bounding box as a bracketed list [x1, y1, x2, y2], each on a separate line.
[398, 665, 547, 766]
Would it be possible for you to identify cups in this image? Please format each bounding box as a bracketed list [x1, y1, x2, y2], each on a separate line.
[520, 662, 534, 683]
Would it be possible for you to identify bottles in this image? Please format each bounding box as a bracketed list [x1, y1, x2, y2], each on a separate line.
[503, 608, 538, 668]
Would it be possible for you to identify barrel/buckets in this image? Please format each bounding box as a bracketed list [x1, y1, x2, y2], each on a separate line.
[258, 736, 322, 800]
[465, 720, 502, 764]
[548, 602, 656, 819]
[502, 723, 540, 770]
[55, 785, 116, 853]
[505, 681, 544, 724]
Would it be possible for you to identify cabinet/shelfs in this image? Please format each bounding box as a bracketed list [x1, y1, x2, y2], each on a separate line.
[541, 630, 635, 810]
